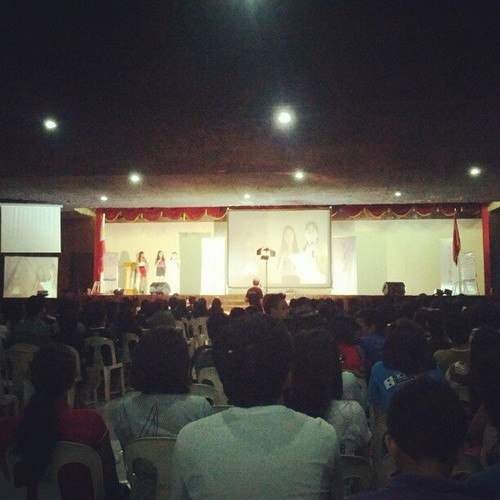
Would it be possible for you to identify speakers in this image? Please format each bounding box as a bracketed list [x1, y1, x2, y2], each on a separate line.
[150, 282, 171, 295]
[382, 282, 405, 296]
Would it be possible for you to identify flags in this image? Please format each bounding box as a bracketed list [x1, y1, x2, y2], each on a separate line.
[453, 211, 461, 266]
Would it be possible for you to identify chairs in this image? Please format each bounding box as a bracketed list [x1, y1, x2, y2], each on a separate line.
[0, 315, 500, 500]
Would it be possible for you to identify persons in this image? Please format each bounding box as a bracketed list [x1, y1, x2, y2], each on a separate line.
[276, 221, 328, 284]
[136, 252, 149, 292]
[245, 278, 264, 313]
[165, 251, 181, 295]
[0, 293, 500, 500]
[155, 250, 166, 282]
